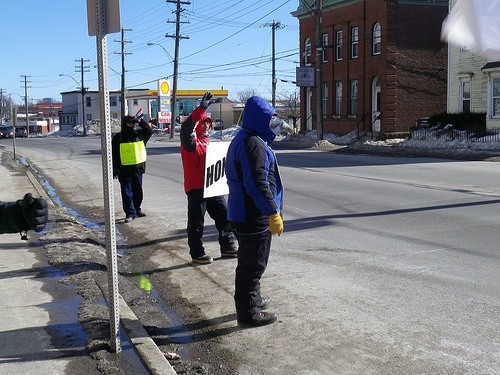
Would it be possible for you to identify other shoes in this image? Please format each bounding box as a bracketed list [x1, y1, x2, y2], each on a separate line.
[125, 217, 133, 223]
[241, 311, 277, 328]
[136, 212, 146, 217]
[257, 297, 271, 311]
[192, 254, 213, 263]
[221, 249, 238, 258]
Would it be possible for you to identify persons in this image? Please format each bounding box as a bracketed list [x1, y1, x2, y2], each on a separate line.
[0, 192, 48, 235]
[111, 115, 154, 224]
[179, 93, 238, 264]
[224, 96, 284, 328]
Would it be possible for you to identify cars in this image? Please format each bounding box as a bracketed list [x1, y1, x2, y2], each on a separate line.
[0, 125, 27, 139]
[212, 118, 224, 130]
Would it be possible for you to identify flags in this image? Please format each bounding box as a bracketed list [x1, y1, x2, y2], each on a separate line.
[136, 108, 144, 119]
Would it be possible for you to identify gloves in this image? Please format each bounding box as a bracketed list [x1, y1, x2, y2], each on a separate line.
[268, 212, 283, 237]
[129, 118, 143, 123]
[113, 169, 120, 179]
[200, 92, 214, 110]
[10, 193, 48, 233]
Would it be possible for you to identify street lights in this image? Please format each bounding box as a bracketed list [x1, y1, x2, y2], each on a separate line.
[146, 42, 179, 138]
[94, 64, 126, 116]
[59, 73, 87, 137]
